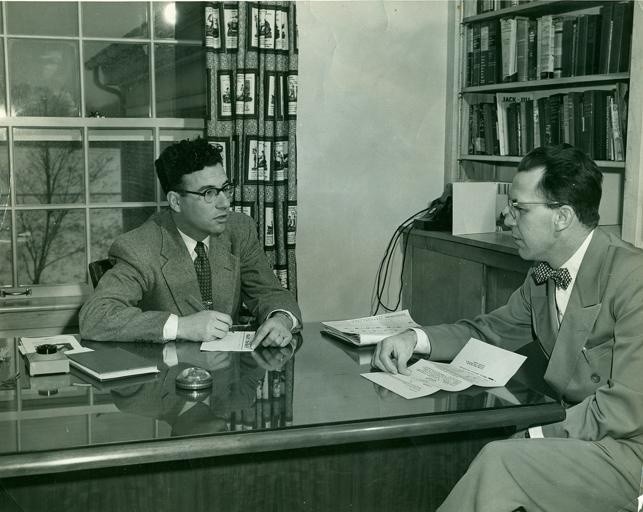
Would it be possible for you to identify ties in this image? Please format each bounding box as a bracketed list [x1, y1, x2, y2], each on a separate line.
[192, 241, 213, 310]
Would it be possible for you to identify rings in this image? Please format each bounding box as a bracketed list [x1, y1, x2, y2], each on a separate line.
[278, 356, 285, 362]
[278, 334, 286, 340]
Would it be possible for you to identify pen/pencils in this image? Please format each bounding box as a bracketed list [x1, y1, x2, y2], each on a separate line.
[190, 294, 235, 334]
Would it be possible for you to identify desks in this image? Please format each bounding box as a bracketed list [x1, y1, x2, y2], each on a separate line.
[0, 319, 565, 512]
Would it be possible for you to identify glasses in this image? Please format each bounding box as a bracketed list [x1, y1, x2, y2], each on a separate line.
[176, 183, 235, 202]
[508, 201, 564, 219]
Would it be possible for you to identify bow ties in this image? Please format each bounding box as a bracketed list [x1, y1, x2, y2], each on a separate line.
[534, 263, 571, 290]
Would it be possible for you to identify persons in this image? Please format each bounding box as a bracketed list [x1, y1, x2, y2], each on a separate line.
[78, 137, 303, 351]
[110, 331, 302, 436]
[371, 142, 643, 512]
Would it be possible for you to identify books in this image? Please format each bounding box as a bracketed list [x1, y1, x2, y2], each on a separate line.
[68, 343, 159, 381]
[465, 0, 634, 162]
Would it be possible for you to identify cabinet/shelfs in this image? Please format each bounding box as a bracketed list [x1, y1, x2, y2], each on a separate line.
[399, 224, 626, 326]
[454, 0, 643, 249]
